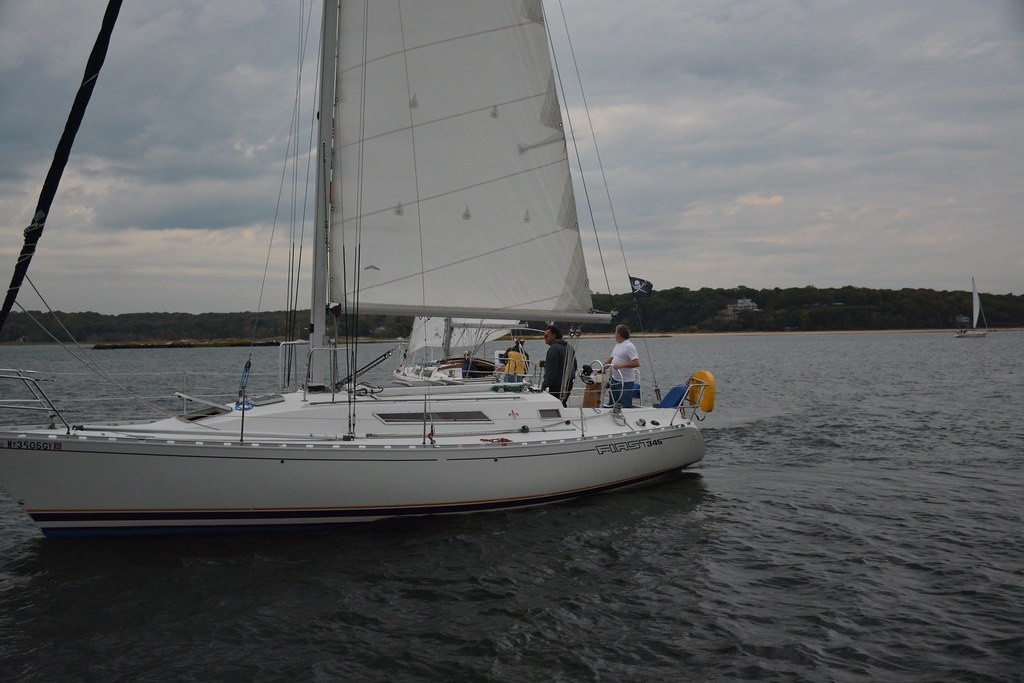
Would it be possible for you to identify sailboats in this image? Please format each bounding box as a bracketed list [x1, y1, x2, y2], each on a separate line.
[953, 277, 988, 337]
[0, 1, 716, 541]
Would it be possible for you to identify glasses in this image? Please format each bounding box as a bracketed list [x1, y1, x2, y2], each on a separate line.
[545, 333, 550, 338]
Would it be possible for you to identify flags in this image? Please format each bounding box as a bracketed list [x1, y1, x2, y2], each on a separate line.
[630, 277, 653, 297]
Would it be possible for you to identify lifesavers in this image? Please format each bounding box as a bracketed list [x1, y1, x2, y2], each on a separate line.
[687, 369, 715, 413]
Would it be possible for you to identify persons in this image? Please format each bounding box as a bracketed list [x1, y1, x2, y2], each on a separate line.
[541, 326, 577, 408]
[501, 337, 529, 383]
[602, 324, 640, 408]
